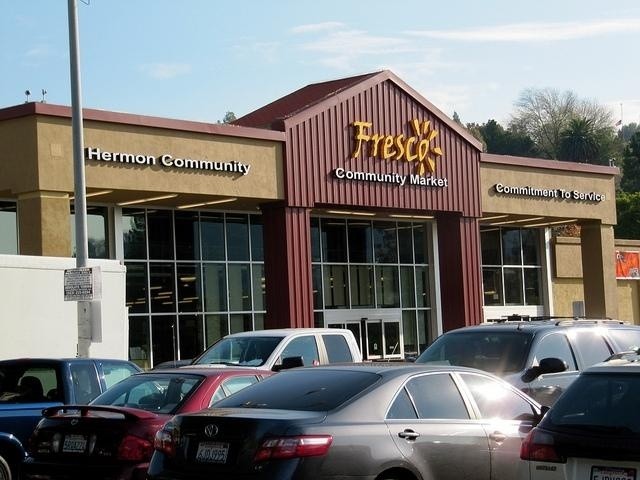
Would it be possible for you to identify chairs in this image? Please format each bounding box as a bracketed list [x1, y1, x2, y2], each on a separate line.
[76, 371, 89, 400]
[20, 376, 43, 399]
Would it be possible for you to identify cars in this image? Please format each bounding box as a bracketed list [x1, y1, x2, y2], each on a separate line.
[181, 327, 362, 370]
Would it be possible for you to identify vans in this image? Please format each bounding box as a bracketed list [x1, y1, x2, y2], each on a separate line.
[414, 313, 640, 406]
